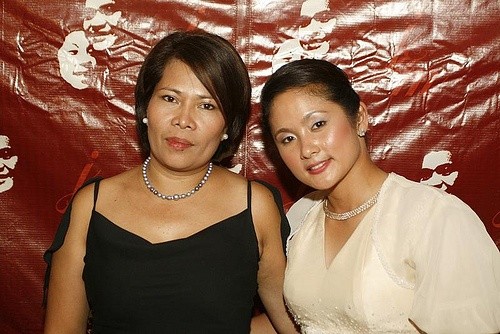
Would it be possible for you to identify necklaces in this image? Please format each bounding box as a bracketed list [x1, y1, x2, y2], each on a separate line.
[143, 156, 213, 200]
[322, 192, 379, 221]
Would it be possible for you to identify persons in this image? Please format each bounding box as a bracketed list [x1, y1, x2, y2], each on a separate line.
[40, 28, 302, 334]
[249, 57, 500, 334]
